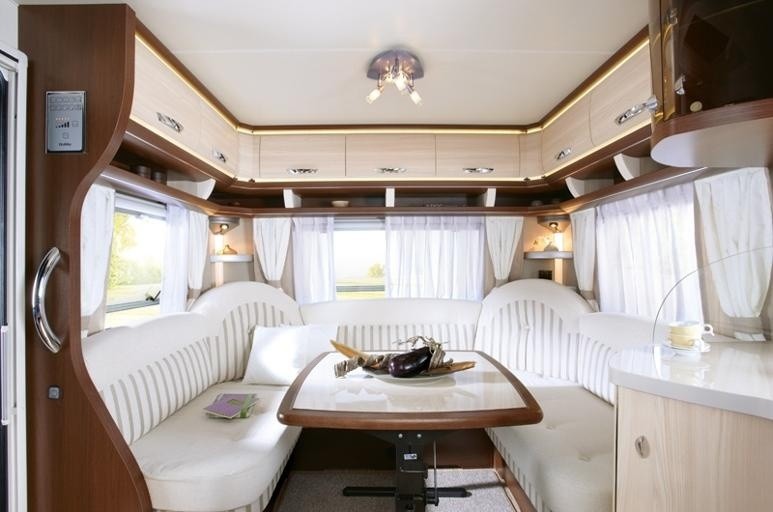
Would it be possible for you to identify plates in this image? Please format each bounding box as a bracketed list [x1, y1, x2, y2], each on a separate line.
[362, 367, 450, 386]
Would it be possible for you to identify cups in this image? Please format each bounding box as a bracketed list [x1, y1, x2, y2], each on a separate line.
[671, 321, 715, 336]
[665, 336, 711, 353]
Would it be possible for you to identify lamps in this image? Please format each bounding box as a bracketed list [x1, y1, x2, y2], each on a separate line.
[365, 49, 423, 106]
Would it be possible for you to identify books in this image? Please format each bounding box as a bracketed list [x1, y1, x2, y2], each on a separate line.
[203, 393, 259, 418]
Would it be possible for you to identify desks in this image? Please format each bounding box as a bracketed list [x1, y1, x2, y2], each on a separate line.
[277, 350, 543, 511]
[607, 340, 768, 512]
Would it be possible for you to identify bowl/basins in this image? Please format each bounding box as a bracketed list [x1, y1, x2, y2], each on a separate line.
[331, 200, 350, 208]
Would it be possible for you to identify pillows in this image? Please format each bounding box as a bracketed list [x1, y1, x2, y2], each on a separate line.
[241, 323, 338, 384]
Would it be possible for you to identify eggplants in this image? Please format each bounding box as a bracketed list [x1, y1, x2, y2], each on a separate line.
[390, 344, 443, 378]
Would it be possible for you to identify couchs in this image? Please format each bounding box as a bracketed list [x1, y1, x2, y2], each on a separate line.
[473, 278, 662, 512]
[81, 281, 304, 512]
[301, 299, 482, 351]
